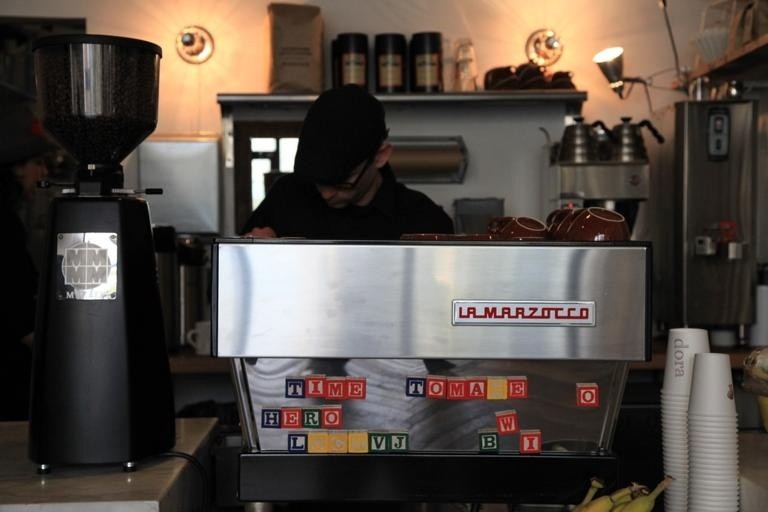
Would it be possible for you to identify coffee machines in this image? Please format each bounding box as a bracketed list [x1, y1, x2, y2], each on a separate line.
[29, 35, 175, 474]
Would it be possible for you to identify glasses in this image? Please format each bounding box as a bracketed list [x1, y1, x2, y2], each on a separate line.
[335, 151, 373, 189]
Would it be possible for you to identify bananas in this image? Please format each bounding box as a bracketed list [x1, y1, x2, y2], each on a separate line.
[571, 475, 675, 512]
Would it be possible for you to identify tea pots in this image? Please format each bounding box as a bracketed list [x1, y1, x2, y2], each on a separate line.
[539, 116, 665, 165]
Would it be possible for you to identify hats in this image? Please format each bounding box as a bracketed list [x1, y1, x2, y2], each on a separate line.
[295, 84, 386, 184]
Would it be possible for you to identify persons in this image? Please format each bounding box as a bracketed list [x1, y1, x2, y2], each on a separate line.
[232, 79, 456, 241]
[0, 116, 46, 422]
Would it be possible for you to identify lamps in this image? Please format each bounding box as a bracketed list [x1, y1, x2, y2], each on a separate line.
[592, 46, 689, 99]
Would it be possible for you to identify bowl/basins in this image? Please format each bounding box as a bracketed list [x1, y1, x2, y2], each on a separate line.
[511, 207, 629, 242]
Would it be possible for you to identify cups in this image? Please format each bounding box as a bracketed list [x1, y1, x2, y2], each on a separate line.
[660, 328, 739, 511]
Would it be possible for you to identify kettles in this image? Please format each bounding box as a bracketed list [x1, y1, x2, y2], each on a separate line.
[186, 322, 211, 356]
